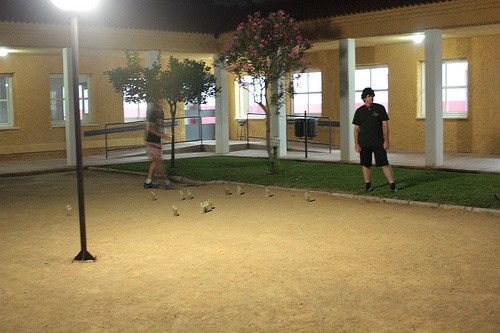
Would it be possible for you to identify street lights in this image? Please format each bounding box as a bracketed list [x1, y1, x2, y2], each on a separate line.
[51, 0, 99, 262]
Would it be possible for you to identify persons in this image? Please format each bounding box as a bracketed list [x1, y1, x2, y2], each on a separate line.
[143, 97, 177, 189]
[353, 88, 398, 193]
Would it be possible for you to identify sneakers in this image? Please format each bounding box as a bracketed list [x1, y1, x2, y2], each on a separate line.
[364, 183, 373, 193]
[165, 180, 177, 188]
[144, 181, 161, 188]
[389, 183, 397, 193]
